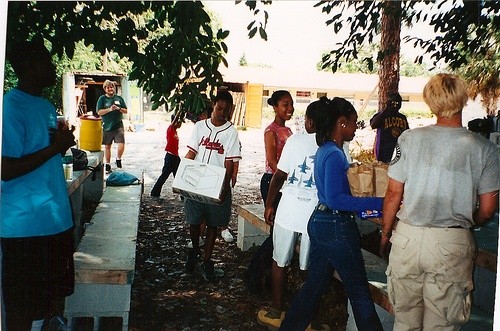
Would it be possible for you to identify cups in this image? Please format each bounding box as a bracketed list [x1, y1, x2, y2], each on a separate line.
[392, 101, 398, 107]
[56, 117, 65, 131]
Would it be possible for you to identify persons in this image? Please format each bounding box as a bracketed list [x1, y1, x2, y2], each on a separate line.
[256, 99, 325, 328]
[279, 96, 386, 330]
[151, 102, 188, 202]
[1, 41, 79, 331]
[381, 76, 500, 330]
[174, 91, 242, 287]
[236, 89, 294, 295]
[370, 93, 410, 163]
[96, 80, 128, 174]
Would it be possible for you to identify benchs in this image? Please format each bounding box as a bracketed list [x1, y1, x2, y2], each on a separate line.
[235, 202, 395, 317]
[64, 169, 146, 331]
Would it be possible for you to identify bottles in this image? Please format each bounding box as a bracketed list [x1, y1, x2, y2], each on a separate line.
[63, 148, 74, 183]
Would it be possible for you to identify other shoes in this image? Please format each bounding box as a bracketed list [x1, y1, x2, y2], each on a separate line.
[256, 309, 281, 331]
[221, 229, 234, 242]
[187, 237, 204, 248]
[106, 164, 111, 174]
[200, 260, 217, 281]
[185, 249, 202, 274]
[116, 160, 122, 168]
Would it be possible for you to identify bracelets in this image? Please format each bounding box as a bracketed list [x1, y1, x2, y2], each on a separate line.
[379, 229, 392, 239]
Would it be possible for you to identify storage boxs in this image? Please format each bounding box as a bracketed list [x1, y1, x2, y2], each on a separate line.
[168, 157, 229, 206]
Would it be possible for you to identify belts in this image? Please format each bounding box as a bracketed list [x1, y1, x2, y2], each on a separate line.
[317, 204, 351, 214]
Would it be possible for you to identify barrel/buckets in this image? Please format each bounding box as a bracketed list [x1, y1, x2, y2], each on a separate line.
[79, 114, 103, 151]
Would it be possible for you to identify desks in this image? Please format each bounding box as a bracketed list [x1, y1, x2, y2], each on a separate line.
[368, 216, 499, 313]
[61, 148, 108, 236]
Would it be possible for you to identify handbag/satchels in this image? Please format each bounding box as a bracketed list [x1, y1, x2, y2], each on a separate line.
[107, 172, 137, 186]
[70, 148, 88, 170]
